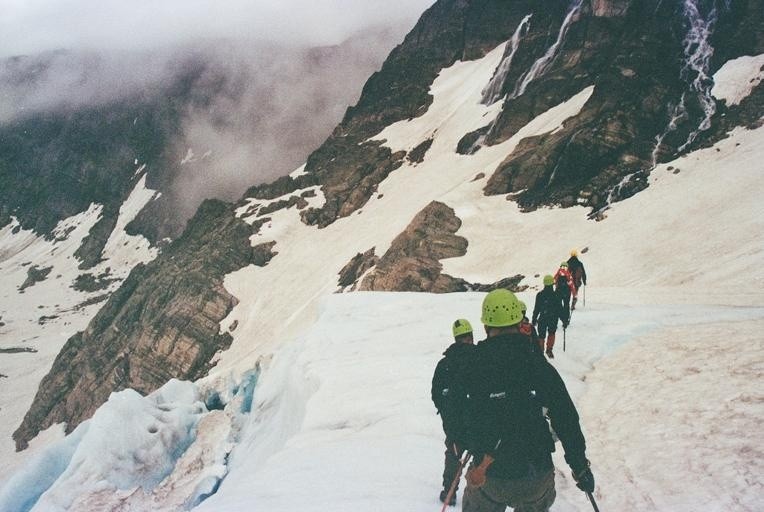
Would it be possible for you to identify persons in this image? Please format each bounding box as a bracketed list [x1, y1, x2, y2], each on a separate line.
[517, 300, 537, 335]
[552, 261, 576, 317]
[430, 315, 477, 505]
[445, 288, 595, 512]
[566, 250, 586, 292]
[530, 273, 565, 358]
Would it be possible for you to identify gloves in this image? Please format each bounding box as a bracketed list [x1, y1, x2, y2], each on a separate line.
[572, 466, 595, 494]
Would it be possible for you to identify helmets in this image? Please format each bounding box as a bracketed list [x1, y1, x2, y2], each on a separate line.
[543, 250, 577, 286]
[480, 289, 527, 327]
[452, 318, 474, 337]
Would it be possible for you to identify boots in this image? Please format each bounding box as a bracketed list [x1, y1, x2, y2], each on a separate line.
[537, 335, 555, 359]
[440, 458, 462, 507]
